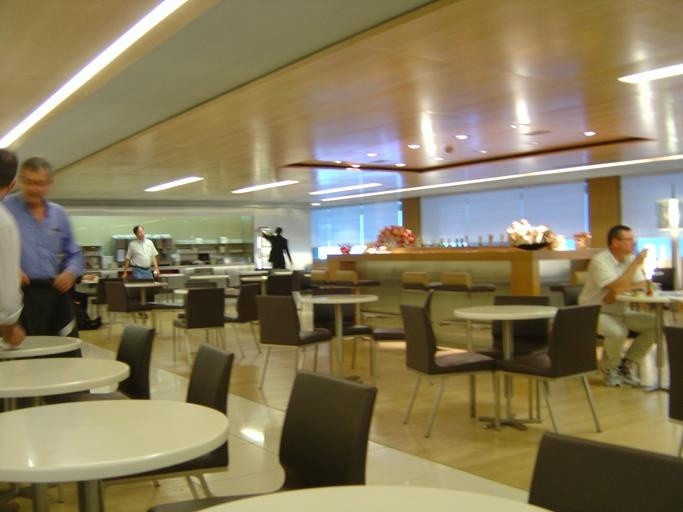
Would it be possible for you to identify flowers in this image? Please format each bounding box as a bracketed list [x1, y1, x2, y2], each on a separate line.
[374, 226, 416, 248]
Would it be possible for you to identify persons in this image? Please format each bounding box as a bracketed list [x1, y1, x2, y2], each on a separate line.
[0, 148, 25, 346]
[261, 227, 292, 268]
[121, 226, 160, 280]
[577, 225, 668, 387]
[1, 158, 87, 358]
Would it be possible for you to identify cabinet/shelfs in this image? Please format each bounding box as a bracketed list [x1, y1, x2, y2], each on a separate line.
[176, 241, 253, 266]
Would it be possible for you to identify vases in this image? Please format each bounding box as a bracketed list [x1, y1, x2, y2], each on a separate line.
[387, 240, 399, 251]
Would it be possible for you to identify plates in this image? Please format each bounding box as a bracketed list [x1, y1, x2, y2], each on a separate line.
[514, 242, 551, 251]
